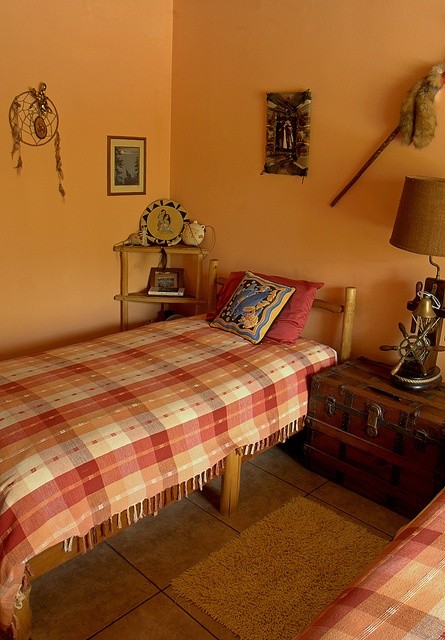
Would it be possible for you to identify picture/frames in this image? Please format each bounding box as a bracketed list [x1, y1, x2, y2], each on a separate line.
[139, 199, 189, 246]
[149, 267, 185, 289]
[263, 90, 311, 177]
[106, 134, 147, 196]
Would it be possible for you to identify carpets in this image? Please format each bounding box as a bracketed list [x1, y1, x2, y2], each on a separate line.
[169, 494, 388, 638]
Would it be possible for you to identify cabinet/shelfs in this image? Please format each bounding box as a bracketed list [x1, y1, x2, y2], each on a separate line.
[112, 244, 208, 331]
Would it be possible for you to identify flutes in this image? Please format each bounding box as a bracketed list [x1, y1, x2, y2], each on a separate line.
[329, 125, 402, 207]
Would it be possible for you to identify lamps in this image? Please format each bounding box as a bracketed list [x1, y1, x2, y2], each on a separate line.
[379, 173, 445, 393]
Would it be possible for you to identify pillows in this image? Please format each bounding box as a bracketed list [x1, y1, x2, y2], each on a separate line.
[210, 270, 296, 345]
[207, 264, 324, 346]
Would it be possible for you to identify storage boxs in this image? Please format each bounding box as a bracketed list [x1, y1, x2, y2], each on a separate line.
[302, 355, 445, 511]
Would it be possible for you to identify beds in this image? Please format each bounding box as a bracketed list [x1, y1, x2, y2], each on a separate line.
[298, 485, 445, 640]
[0, 258, 360, 639]
